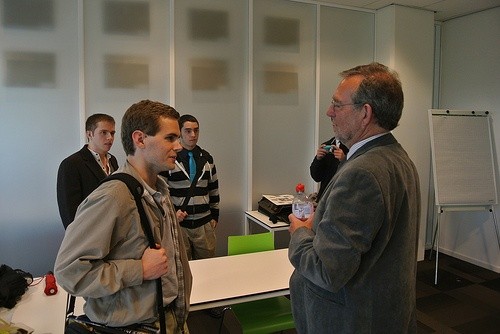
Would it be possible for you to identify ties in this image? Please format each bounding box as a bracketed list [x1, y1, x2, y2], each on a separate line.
[188, 152, 196, 182]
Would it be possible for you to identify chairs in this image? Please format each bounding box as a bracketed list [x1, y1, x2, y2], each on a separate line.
[218, 232, 291, 334]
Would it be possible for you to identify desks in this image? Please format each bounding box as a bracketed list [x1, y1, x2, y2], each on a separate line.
[245, 210, 290, 235]
[0, 247, 294, 334]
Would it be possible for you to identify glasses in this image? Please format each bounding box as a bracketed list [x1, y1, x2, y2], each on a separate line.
[331, 100, 364, 110]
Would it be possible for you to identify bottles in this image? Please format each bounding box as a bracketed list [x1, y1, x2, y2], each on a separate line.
[292, 183, 314, 221]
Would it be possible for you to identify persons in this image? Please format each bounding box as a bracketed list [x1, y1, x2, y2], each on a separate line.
[309, 127, 350, 212]
[56, 113, 119, 234]
[287, 62, 417, 334]
[156, 114, 222, 318]
[54, 99, 193, 334]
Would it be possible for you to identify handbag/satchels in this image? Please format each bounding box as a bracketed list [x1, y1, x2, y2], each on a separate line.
[64, 314, 167, 334]
[0, 264, 33, 309]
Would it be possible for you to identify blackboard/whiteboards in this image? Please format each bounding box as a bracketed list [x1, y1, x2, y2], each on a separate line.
[428, 108, 498, 206]
